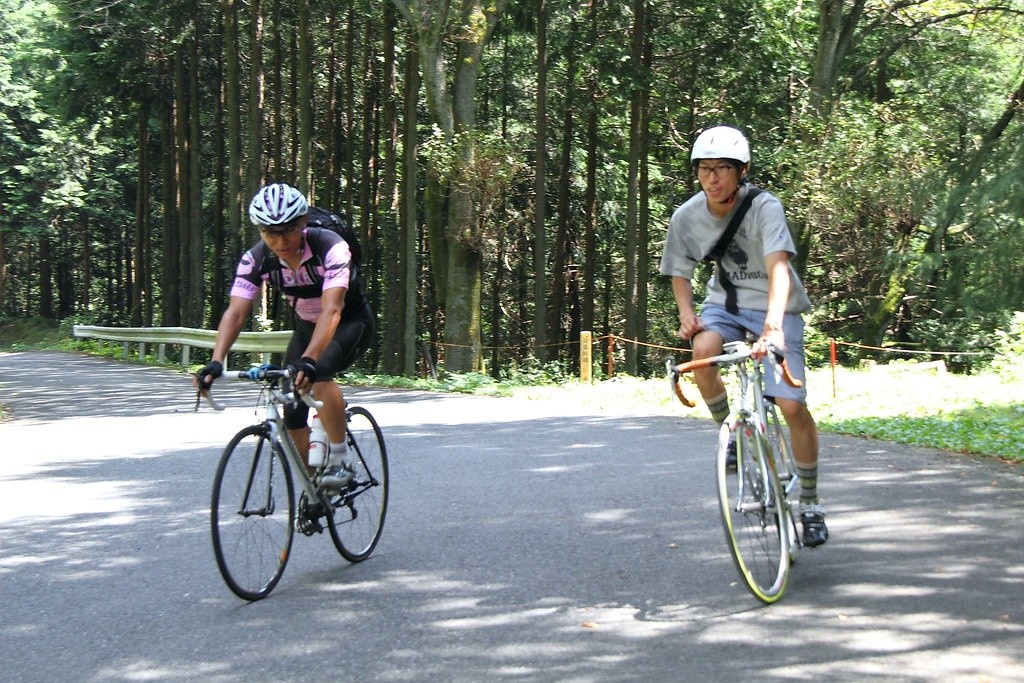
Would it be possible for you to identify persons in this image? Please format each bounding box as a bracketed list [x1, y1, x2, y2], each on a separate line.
[193, 181, 375, 530]
[659, 127, 830, 549]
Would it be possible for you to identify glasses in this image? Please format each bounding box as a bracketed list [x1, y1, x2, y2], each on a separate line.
[260, 221, 302, 237]
[694, 165, 741, 176]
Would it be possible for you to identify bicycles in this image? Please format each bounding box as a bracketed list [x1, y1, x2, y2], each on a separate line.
[669, 330, 811, 605]
[194, 364, 391, 602]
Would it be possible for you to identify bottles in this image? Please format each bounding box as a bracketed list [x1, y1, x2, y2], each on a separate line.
[307, 413, 330, 467]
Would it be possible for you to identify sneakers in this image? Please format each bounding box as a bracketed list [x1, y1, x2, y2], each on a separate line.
[714, 440, 737, 470]
[798, 501, 828, 547]
[320, 462, 354, 488]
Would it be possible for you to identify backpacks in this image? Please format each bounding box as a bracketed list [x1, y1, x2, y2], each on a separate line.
[268, 205, 361, 296]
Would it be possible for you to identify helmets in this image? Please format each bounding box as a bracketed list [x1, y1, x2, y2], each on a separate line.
[249, 183, 308, 228]
[690, 126, 751, 166]
